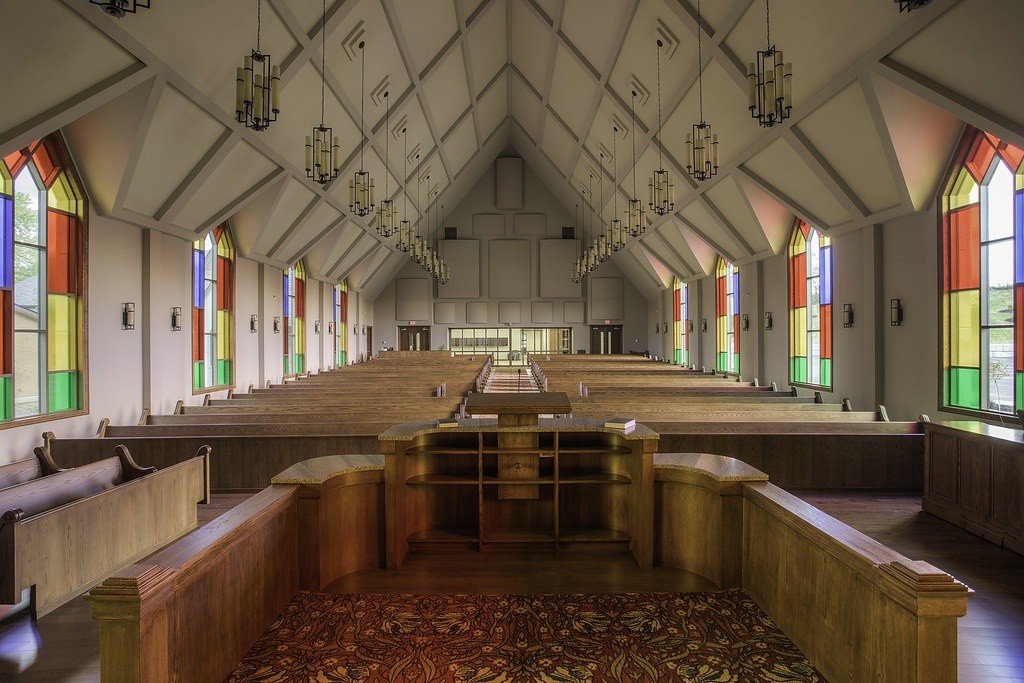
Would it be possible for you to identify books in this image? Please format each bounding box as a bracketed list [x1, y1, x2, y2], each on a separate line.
[604, 417, 636, 429]
[436, 418, 459, 428]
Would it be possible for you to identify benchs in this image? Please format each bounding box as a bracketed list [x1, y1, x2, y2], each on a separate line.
[1, 444, 158, 521]
[1, 444, 75, 492]
[38, 344, 932, 500]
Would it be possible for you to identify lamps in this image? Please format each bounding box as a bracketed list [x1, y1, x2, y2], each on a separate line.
[232, 1, 452, 287]
[569, 0, 792, 284]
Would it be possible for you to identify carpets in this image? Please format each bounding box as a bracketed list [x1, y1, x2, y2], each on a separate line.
[220, 587, 827, 683]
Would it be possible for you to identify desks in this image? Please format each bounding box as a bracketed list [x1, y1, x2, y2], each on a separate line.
[88, 387, 974, 681]
[920, 417, 1024, 554]
